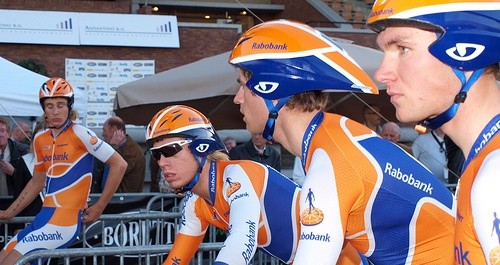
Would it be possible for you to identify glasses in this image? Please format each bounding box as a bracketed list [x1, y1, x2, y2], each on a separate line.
[149, 137, 193, 161]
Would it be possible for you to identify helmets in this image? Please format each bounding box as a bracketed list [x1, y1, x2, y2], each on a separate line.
[228, 20, 380, 100]
[145, 105, 229, 156]
[39, 77, 74, 107]
[366, 0, 500, 71]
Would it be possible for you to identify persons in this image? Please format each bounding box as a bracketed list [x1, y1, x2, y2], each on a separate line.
[367, 0, 500, 265]
[0, 115, 145, 265]
[222, 133, 281, 170]
[228, 18, 458, 265]
[0, 77, 129, 265]
[144, 105, 369, 265]
[359, 103, 461, 196]
